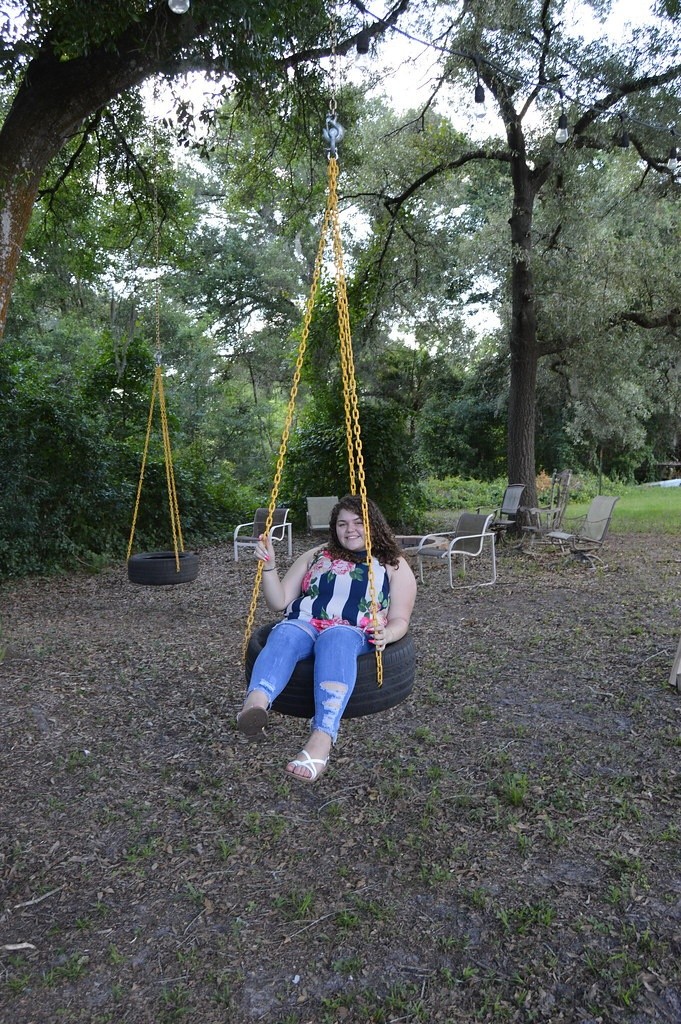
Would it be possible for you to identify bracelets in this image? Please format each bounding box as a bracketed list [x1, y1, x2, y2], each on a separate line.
[262, 566, 277, 572]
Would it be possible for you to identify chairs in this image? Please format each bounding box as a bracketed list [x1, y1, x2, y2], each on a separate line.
[548, 493, 620, 566]
[233, 507, 294, 567]
[475, 483, 526, 550]
[515, 467, 574, 552]
[415, 511, 500, 590]
[304, 495, 342, 536]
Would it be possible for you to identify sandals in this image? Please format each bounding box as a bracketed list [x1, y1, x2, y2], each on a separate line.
[283, 749, 330, 782]
[236, 706, 267, 744]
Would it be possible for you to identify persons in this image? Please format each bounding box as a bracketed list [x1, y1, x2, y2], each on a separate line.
[238, 496, 418, 783]
